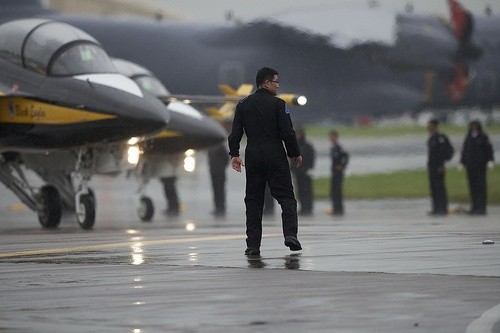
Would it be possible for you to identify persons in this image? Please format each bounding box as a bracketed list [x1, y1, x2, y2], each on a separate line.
[263, 182, 274, 215]
[329, 131, 348, 216]
[208, 144, 231, 216]
[160, 176, 180, 217]
[289, 127, 315, 216]
[425, 119, 454, 217]
[227, 67, 302, 257]
[460, 121, 495, 215]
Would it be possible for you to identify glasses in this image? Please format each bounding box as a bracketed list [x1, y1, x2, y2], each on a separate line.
[272, 80, 279, 83]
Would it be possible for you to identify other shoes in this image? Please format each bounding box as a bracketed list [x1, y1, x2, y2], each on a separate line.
[430, 209, 447, 215]
[245, 246, 260, 255]
[467, 207, 485, 215]
[285, 235, 302, 251]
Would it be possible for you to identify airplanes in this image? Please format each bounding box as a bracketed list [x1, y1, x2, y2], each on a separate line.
[107, 54, 308, 223]
[0, 17, 170, 230]
